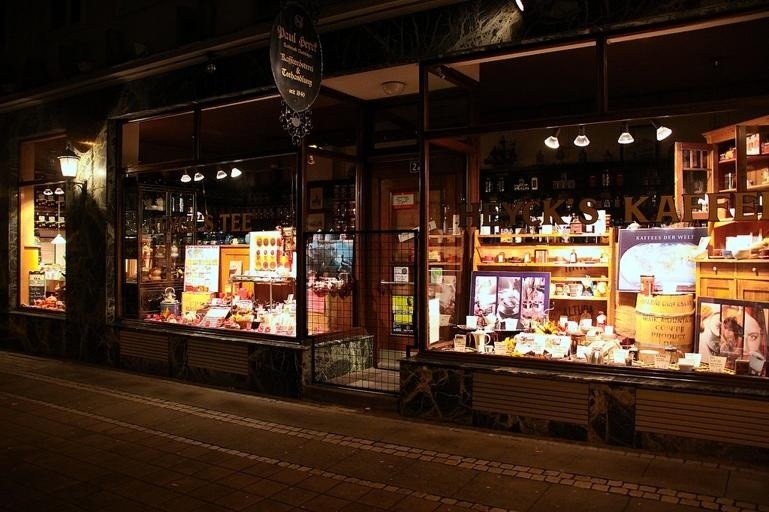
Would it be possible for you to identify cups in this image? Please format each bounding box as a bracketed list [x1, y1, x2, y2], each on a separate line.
[749, 351, 766, 375]
[664, 345, 683, 364]
[470, 328, 490, 355]
[503, 299, 519, 310]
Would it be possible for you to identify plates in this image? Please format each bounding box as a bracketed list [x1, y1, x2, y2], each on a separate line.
[498, 302, 519, 316]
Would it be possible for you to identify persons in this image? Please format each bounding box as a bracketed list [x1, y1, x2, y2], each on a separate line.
[742, 307, 768, 361]
[719, 315, 743, 370]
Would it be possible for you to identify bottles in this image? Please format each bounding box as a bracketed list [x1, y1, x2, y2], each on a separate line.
[640, 274, 655, 294]
[569, 249, 577, 263]
[596, 311, 607, 327]
[580, 310, 592, 327]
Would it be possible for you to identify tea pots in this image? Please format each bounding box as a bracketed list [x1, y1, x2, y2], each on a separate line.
[584, 346, 610, 365]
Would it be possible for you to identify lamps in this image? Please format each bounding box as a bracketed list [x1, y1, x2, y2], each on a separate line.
[57, 143, 88, 200]
[180, 164, 243, 183]
[543, 120, 673, 150]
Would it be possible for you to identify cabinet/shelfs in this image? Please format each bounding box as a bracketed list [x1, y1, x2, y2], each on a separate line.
[121, 183, 198, 318]
[674, 113, 768, 301]
[470, 229, 612, 330]
